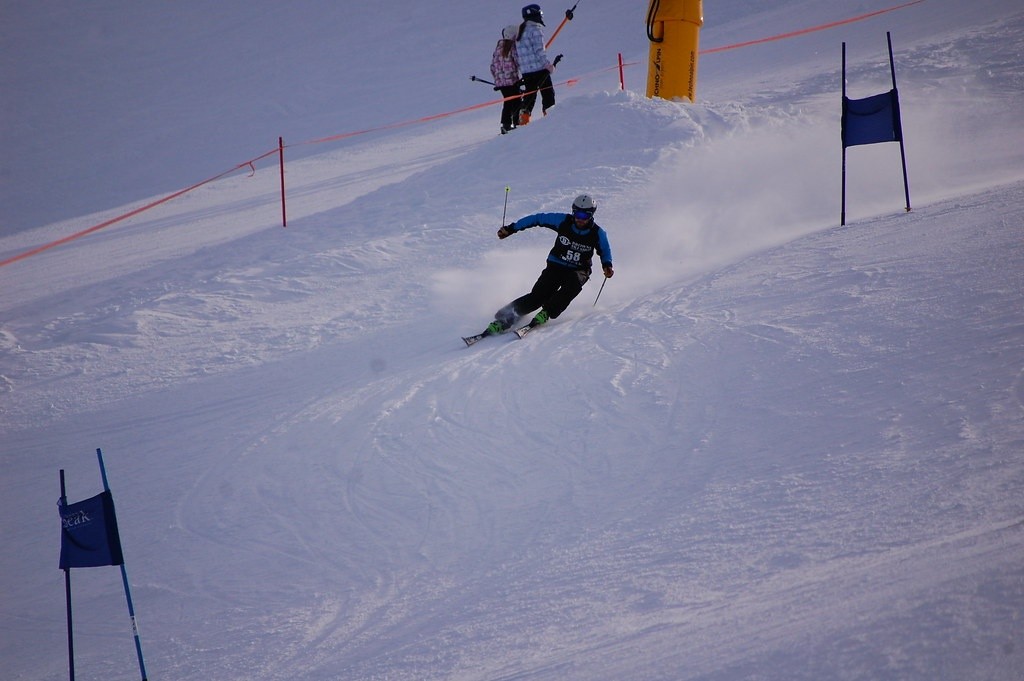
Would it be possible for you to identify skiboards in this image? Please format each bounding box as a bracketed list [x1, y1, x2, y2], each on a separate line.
[461, 308, 549, 349]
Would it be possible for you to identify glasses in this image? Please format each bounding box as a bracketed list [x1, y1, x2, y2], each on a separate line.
[575, 213, 590, 220]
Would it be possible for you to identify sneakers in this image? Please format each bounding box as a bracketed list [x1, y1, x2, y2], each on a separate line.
[501, 123, 517, 134]
[518, 109, 531, 125]
[488, 321, 502, 335]
[535, 310, 549, 324]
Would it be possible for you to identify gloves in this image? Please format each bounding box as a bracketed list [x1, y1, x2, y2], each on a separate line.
[547, 64, 556, 74]
[497, 223, 516, 239]
[602, 262, 614, 278]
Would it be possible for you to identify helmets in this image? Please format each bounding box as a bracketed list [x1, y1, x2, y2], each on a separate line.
[502, 25, 519, 41]
[572, 192, 597, 217]
[522, 4, 544, 20]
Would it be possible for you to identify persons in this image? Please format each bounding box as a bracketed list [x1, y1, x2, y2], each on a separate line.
[490, 25, 520, 134]
[517, 4, 557, 126]
[493, 190, 615, 334]
